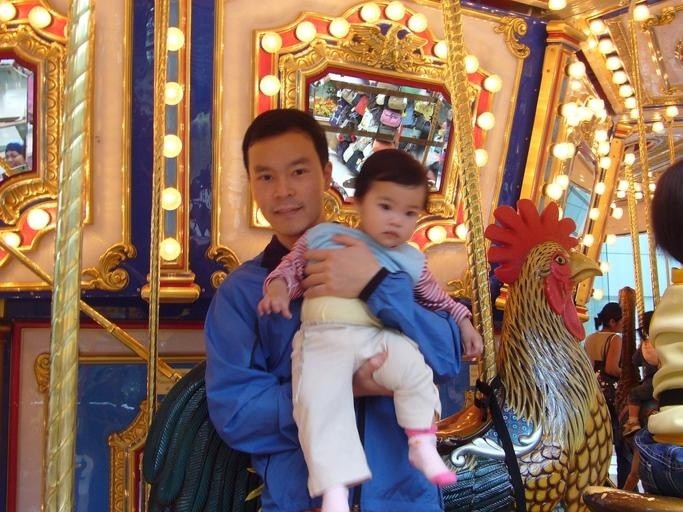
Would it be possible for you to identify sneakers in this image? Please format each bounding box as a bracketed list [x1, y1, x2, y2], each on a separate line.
[622, 418, 641, 438]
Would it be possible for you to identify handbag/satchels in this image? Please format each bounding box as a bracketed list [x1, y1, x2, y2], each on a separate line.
[328, 88, 432, 178]
[594, 333, 623, 408]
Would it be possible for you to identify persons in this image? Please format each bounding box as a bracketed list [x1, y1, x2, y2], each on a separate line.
[202, 107, 464, 511]
[0, 142, 26, 182]
[630, 156, 682, 501]
[581, 300, 639, 493]
[620, 309, 658, 440]
[255, 148, 486, 511]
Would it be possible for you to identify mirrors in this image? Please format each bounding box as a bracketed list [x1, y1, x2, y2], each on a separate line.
[1, 48, 45, 192]
[309, 72, 453, 204]
